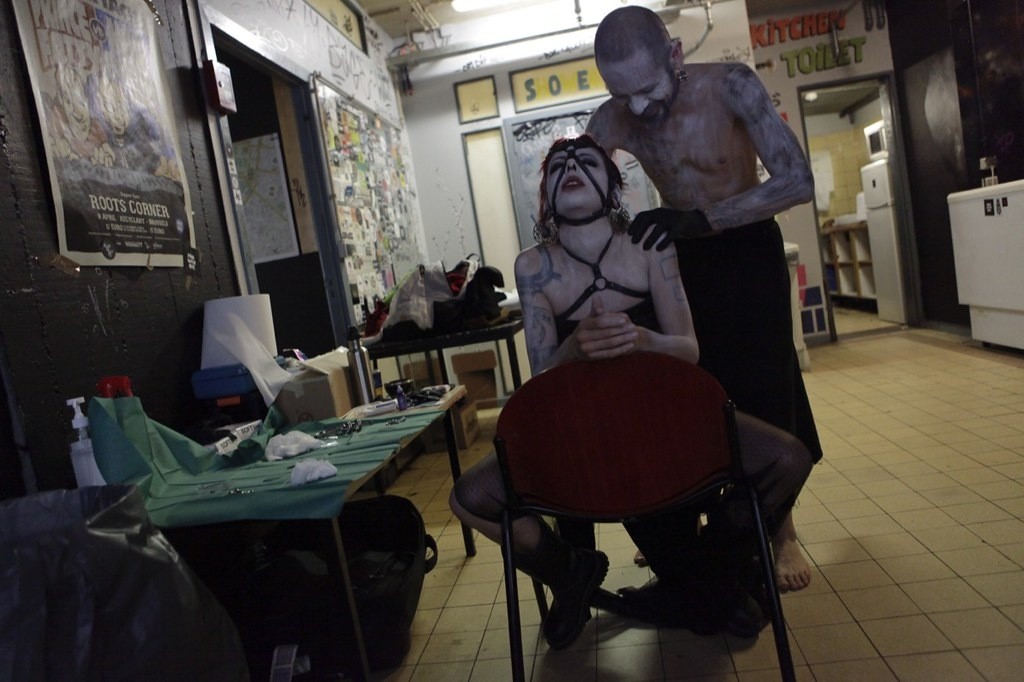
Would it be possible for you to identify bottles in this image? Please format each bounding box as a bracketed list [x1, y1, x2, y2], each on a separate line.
[396, 385, 407, 411]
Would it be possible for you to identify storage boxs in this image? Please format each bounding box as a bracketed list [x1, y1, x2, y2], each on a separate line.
[183, 356, 287, 433]
[279, 345, 357, 426]
[399, 349, 496, 450]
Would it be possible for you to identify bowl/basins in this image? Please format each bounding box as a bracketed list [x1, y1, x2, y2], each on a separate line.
[384, 377, 415, 399]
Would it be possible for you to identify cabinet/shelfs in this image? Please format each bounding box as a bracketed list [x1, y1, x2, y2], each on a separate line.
[822, 220, 876, 299]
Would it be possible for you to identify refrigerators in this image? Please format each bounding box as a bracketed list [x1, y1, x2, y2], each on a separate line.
[860, 159, 907, 324]
[946, 179, 1024, 352]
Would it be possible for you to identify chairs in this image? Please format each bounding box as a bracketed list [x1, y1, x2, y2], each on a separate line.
[491, 351, 797, 682]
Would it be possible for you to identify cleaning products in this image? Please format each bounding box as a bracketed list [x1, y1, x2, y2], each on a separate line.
[66, 396, 108, 487]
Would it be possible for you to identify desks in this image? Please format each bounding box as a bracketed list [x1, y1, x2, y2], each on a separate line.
[140, 386, 478, 682]
[366, 311, 525, 392]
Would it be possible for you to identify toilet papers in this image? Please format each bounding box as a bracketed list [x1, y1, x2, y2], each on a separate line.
[201, 292, 293, 409]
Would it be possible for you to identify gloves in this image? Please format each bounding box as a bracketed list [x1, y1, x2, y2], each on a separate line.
[626, 208, 710, 251]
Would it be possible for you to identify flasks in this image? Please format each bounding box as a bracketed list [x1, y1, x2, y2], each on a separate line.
[346, 326, 375, 406]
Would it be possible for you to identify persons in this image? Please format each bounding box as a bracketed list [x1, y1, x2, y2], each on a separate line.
[582, 5, 826, 591]
[447, 133, 809, 652]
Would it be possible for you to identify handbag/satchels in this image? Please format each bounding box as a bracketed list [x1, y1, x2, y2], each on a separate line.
[163, 493, 437, 675]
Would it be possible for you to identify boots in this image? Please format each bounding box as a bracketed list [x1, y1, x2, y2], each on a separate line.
[680, 507, 762, 639]
[510, 515, 609, 650]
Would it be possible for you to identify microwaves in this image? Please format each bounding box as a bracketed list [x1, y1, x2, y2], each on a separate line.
[863, 120, 890, 161]
